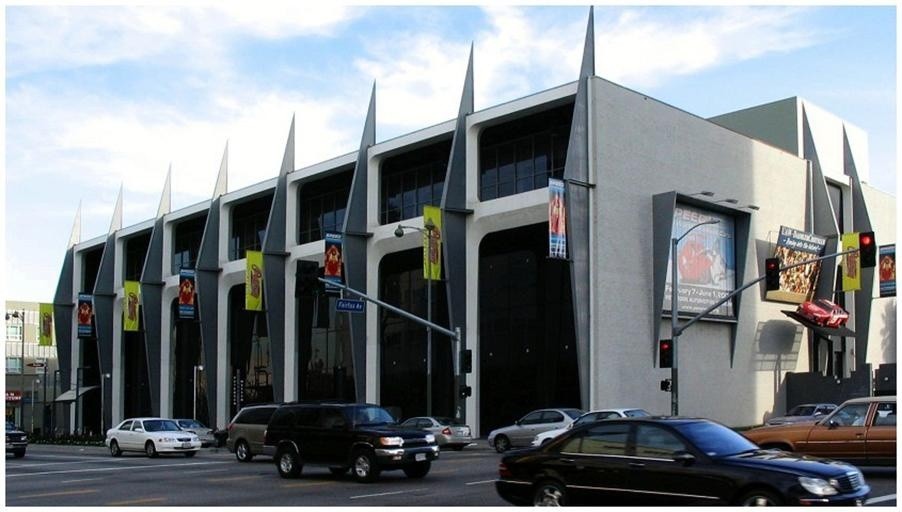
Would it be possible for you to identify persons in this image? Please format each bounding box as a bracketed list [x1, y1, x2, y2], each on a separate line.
[549, 192, 565, 235]
[880, 254, 895, 280]
[324, 245, 343, 277]
[427, 222, 441, 263]
[77, 302, 94, 325]
[846, 246, 859, 278]
[775, 246, 820, 295]
[248, 263, 263, 297]
[41, 312, 54, 339]
[126, 292, 138, 319]
[177, 279, 195, 304]
[310, 348, 325, 374]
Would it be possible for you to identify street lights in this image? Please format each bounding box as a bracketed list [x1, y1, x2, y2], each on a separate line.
[5, 309, 25, 415]
[392, 219, 438, 403]
[668, 216, 720, 417]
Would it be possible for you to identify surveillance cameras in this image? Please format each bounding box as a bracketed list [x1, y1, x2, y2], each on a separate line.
[6, 315, 10, 320]
[394, 229, 404, 237]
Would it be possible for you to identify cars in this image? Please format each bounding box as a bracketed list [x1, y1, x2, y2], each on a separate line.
[224, 404, 471, 484]
[104, 415, 214, 457]
[5, 417, 29, 457]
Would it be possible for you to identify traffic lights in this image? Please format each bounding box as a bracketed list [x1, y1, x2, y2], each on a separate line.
[858, 235, 879, 265]
[764, 260, 779, 289]
[658, 339, 673, 369]
[458, 350, 473, 376]
